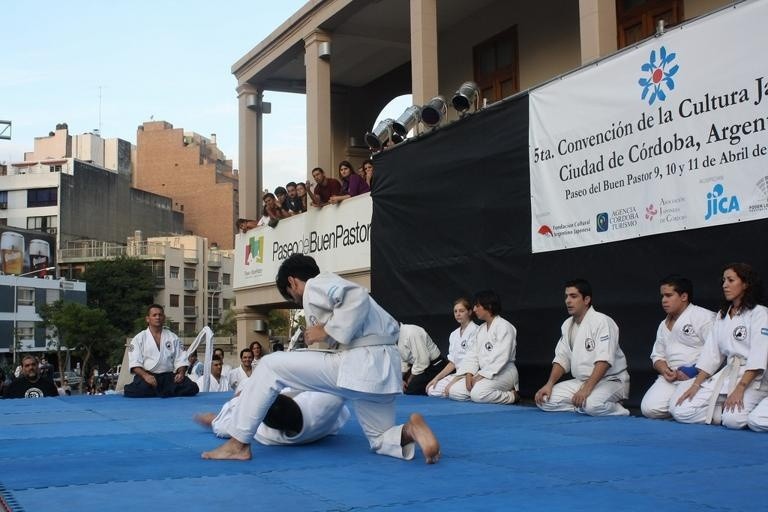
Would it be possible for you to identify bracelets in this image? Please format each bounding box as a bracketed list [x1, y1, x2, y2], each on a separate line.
[692, 382, 701, 387]
[738, 381, 747, 388]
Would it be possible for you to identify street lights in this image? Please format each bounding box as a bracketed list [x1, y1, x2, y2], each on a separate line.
[12, 267, 56, 364]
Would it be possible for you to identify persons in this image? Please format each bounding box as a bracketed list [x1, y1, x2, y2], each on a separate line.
[396, 321, 447, 396]
[328, 160, 369, 203]
[62, 380, 72, 396]
[274, 186, 295, 217]
[425, 296, 480, 400]
[194, 377, 351, 446]
[449, 290, 522, 405]
[386, 135, 403, 148]
[671, 262, 768, 430]
[195, 354, 228, 393]
[640, 278, 717, 425]
[185, 348, 204, 381]
[12, 364, 22, 378]
[87, 366, 110, 395]
[200, 252, 442, 464]
[285, 181, 302, 213]
[237, 218, 258, 233]
[3, 355, 59, 399]
[39, 357, 55, 378]
[362, 159, 374, 191]
[214, 347, 232, 377]
[55, 381, 66, 396]
[123, 303, 199, 396]
[534, 278, 632, 416]
[747, 396, 768, 432]
[250, 341, 263, 368]
[305, 167, 342, 205]
[226, 348, 256, 392]
[0, 367, 10, 399]
[262, 193, 284, 220]
[296, 182, 306, 213]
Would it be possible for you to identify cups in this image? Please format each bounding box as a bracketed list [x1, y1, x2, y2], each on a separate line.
[29, 239, 51, 277]
[0, 232, 25, 275]
